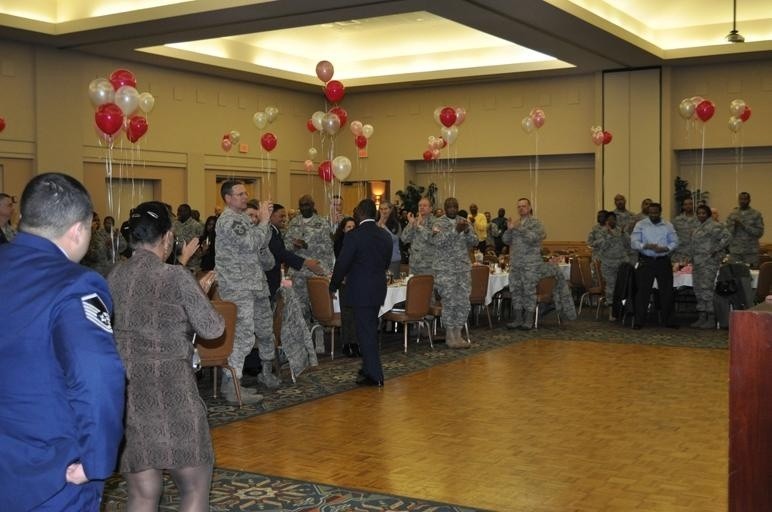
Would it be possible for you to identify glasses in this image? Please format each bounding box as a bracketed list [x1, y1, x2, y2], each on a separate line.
[231, 192, 251, 198]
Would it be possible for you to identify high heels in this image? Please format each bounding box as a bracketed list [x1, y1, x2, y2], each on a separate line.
[633, 321, 643, 329]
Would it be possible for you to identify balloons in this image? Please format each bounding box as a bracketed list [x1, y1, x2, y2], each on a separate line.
[740, 106, 751, 122]
[440, 126, 458, 144]
[521, 117, 534, 134]
[350, 120, 363, 135]
[125, 130, 139, 142]
[0, 117, 7, 132]
[434, 106, 446, 125]
[128, 116, 148, 136]
[221, 139, 231, 152]
[96, 104, 122, 134]
[115, 86, 139, 117]
[690, 96, 703, 119]
[329, 107, 347, 129]
[433, 150, 440, 157]
[318, 160, 335, 183]
[223, 133, 233, 144]
[428, 143, 435, 149]
[362, 126, 374, 137]
[93, 122, 122, 144]
[729, 99, 745, 117]
[308, 148, 318, 161]
[265, 105, 279, 123]
[229, 130, 240, 144]
[529, 108, 545, 129]
[109, 69, 137, 92]
[88, 78, 114, 108]
[604, 131, 612, 145]
[355, 135, 367, 149]
[697, 100, 715, 121]
[434, 138, 444, 149]
[252, 111, 268, 130]
[428, 136, 435, 142]
[440, 107, 456, 127]
[307, 119, 318, 132]
[332, 156, 351, 181]
[262, 132, 277, 152]
[316, 61, 334, 82]
[304, 160, 314, 172]
[423, 151, 432, 160]
[592, 133, 604, 146]
[140, 92, 155, 111]
[453, 107, 466, 127]
[728, 116, 742, 133]
[325, 81, 345, 103]
[678, 99, 695, 119]
[321, 112, 340, 135]
[590, 125, 601, 135]
[311, 111, 325, 131]
[439, 136, 448, 148]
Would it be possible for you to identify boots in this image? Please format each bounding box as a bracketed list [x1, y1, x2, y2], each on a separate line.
[691, 311, 707, 328]
[410, 323, 431, 337]
[259, 361, 282, 389]
[220, 373, 257, 398]
[444, 326, 471, 348]
[519, 310, 535, 331]
[225, 379, 264, 406]
[507, 308, 525, 329]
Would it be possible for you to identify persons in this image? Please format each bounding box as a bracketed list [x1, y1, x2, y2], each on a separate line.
[286, 195, 334, 285]
[199, 216, 217, 269]
[330, 199, 395, 386]
[100, 219, 125, 261]
[691, 205, 732, 329]
[432, 198, 478, 350]
[503, 199, 545, 329]
[589, 210, 607, 265]
[727, 193, 764, 272]
[613, 195, 633, 222]
[211, 180, 283, 403]
[85, 213, 108, 272]
[375, 200, 401, 278]
[596, 213, 629, 320]
[170, 203, 202, 269]
[327, 197, 350, 229]
[258, 204, 326, 305]
[103, 201, 223, 511]
[634, 198, 657, 222]
[483, 213, 500, 249]
[403, 201, 438, 275]
[0, 173, 125, 511]
[493, 209, 509, 254]
[467, 205, 488, 251]
[630, 204, 679, 329]
[0, 195, 21, 242]
[673, 198, 703, 265]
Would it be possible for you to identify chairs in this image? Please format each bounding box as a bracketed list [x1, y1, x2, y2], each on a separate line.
[754, 263, 772, 305]
[471, 265, 493, 328]
[378, 276, 433, 352]
[575, 259, 602, 320]
[195, 302, 243, 408]
[306, 278, 343, 360]
[565, 258, 587, 314]
[271, 300, 283, 378]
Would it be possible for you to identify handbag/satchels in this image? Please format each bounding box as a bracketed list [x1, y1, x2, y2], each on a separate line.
[715, 263, 736, 296]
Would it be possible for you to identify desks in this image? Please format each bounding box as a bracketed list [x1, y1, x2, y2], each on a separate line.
[634, 262, 760, 299]
[331, 271, 414, 316]
[483, 263, 509, 305]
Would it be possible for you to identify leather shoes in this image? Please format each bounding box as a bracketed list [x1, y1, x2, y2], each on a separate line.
[356, 374, 385, 389]
[343, 341, 361, 359]
[665, 321, 676, 329]
